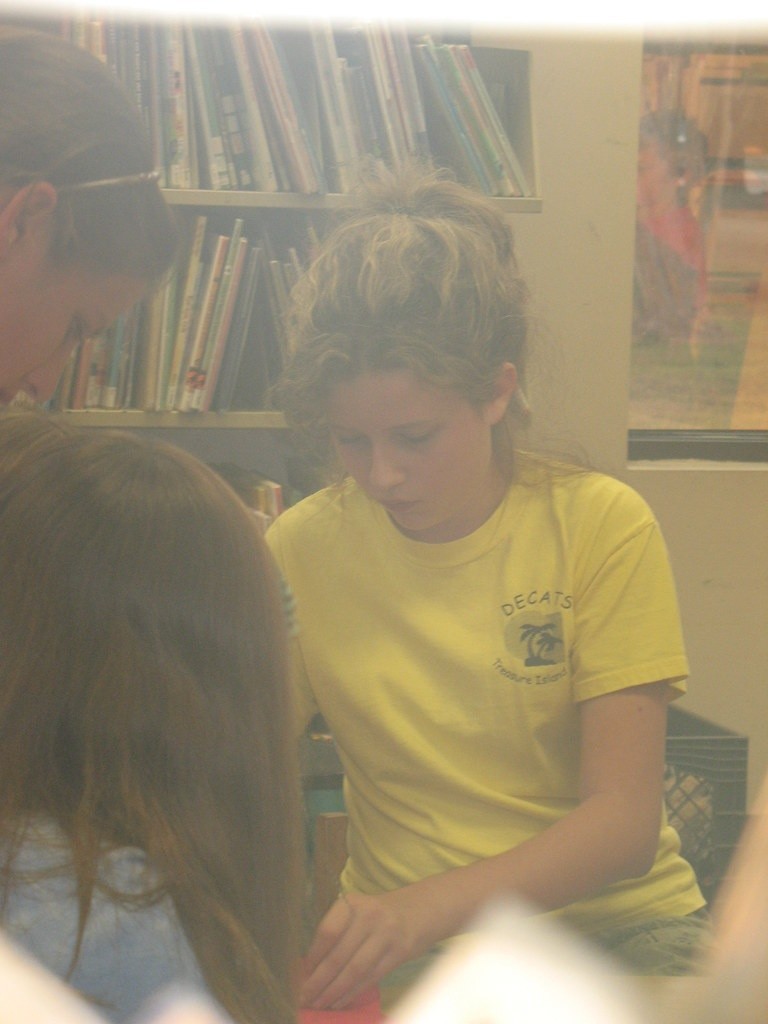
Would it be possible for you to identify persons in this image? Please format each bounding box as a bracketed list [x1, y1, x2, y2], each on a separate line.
[0, 433, 311, 1023]
[0, 26, 173, 405]
[261, 183, 707, 1010]
[635, 111, 711, 351]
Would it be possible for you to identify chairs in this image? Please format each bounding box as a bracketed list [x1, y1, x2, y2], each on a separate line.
[592, 703, 747, 973]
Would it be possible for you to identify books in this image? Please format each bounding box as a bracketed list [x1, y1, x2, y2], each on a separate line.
[12, 22, 530, 412]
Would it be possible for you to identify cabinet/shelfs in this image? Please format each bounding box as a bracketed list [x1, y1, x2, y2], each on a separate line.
[0, 8, 540, 429]
